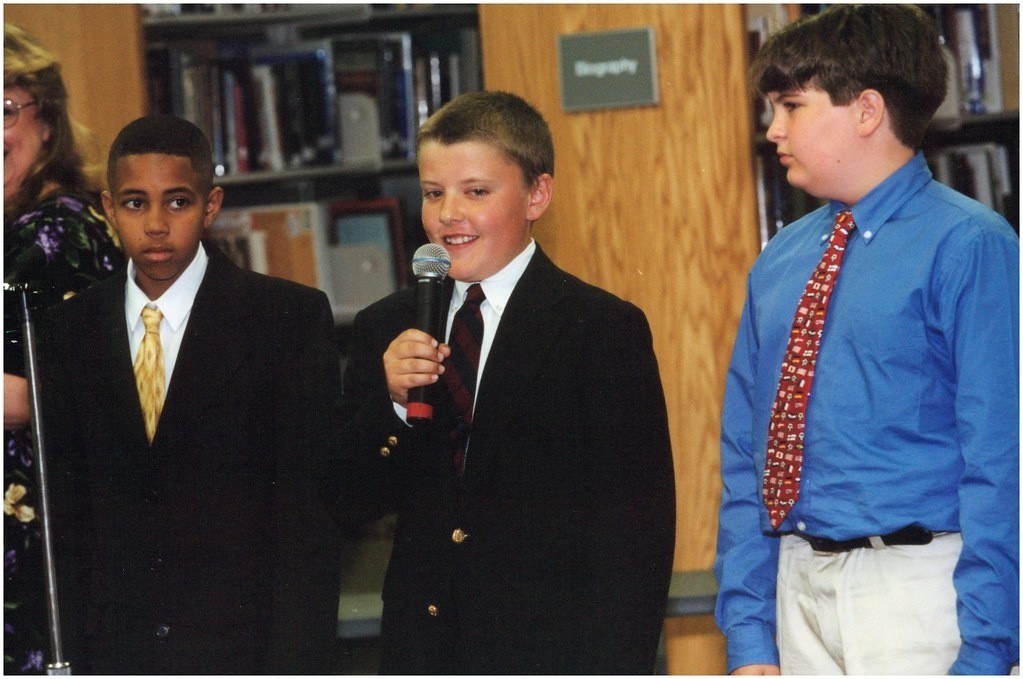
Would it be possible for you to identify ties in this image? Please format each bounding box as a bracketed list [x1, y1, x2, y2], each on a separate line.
[761, 210, 854, 530]
[438, 284, 485, 505]
[134, 305, 168, 441]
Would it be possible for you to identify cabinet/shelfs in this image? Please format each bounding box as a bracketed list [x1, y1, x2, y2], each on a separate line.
[480, 3, 1020, 676]
[3, 3, 486, 646]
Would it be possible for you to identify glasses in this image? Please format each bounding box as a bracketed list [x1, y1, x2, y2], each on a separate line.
[4, 98, 38, 129]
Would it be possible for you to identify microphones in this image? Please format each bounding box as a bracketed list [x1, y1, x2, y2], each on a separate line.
[406, 244, 453, 428]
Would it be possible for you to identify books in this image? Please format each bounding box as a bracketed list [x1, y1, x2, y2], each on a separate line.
[743, 4, 1019, 260]
[136, 3, 485, 319]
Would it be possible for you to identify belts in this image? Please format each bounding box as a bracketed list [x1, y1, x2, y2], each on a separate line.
[792, 523, 938, 553]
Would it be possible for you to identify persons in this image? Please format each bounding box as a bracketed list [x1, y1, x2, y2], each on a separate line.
[3, 22, 127, 675]
[42, 113, 337, 675]
[340, 89, 678, 675]
[712, 4, 1020, 676]
